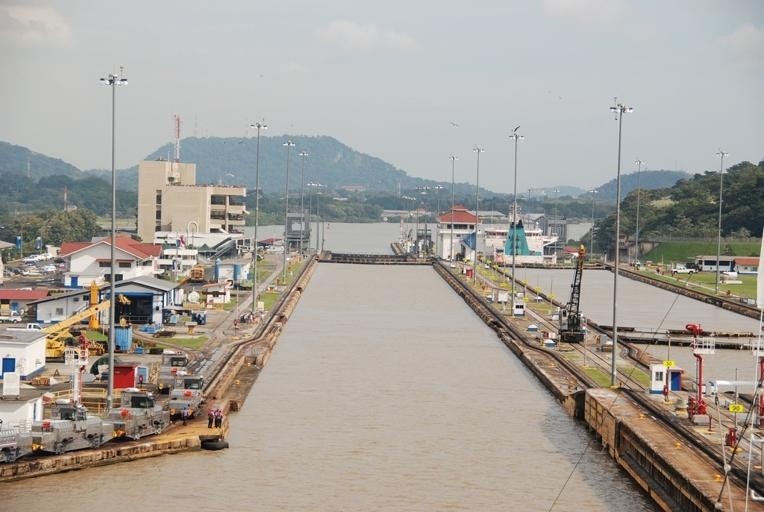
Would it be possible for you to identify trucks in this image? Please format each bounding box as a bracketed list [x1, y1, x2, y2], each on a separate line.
[0, 311, 22, 324]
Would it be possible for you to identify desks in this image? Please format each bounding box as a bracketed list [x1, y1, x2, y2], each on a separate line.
[185, 321, 197, 335]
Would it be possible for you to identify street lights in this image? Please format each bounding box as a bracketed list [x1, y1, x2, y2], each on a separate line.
[609, 104, 633, 386]
[0, 227, 5, 229]
[509, 133, 525, 316]
[40, 225, 52, 254]
[99, 73, 128, 408]
[250, 122, 268, 313]
[449, 155, 459, 261]
[634, 160, 645, 270]
[472, 146, 486, 283]
[283, 141, 296, 284]
[716, 151, 729, 292]
[20, 222, 30, 258]
[297, 150, 322, 254]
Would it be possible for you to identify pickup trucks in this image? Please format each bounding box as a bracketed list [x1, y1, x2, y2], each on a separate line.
[6, 323, 42, 331]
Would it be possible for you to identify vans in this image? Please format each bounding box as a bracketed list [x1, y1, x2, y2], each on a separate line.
[720, 272, 737, 280]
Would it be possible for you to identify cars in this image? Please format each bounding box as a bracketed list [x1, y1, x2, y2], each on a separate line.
[3, 252, 64, 275]
[671, 267, 696, 274]
[631, 259, 640, 266]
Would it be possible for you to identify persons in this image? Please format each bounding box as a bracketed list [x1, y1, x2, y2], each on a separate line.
[208, 408, 222, 428]
[182, 406, 188, 426]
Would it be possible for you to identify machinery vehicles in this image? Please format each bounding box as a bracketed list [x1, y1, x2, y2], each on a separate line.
[0, 349, 204, 467]
[559, 245, 587, 343]
[38, 294, 131, 362]
